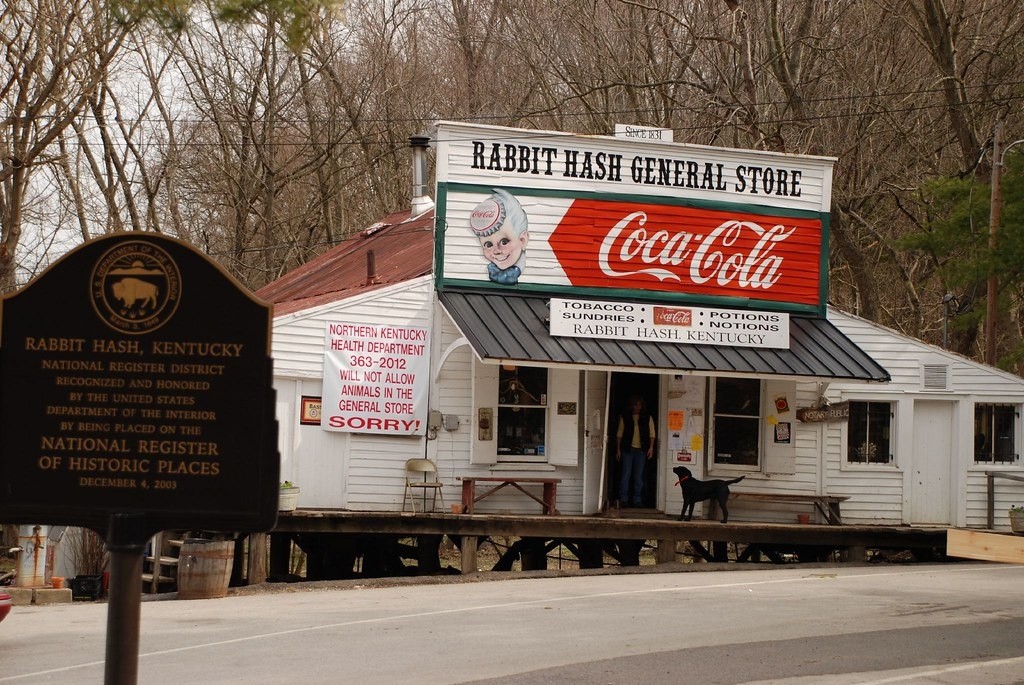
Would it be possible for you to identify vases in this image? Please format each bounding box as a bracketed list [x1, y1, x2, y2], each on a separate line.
[858, 455, 872, 462]
[278, 487, 300, 511]
[52, 576, 65, 588]
[1009, 511, 1024, 532]
[798, 514, 810, 524]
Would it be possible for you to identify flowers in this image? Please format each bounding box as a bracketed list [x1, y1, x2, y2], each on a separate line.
[279, 480, 294, 489]
[1008, 505, 1024, 512]
[857, 443, 877, 457]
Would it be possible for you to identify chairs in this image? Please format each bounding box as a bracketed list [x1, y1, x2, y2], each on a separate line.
[402, 458, 446, 514]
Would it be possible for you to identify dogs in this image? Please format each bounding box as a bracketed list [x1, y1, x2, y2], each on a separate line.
[672, 464, 747, 524]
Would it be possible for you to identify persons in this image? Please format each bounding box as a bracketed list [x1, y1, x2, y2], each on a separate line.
[616, 398, 656, 509]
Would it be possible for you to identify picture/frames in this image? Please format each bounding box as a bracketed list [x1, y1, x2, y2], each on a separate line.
[301, 395, 322, 425]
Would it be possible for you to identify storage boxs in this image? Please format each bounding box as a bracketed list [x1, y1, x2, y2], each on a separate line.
[72, 574, 104, 601]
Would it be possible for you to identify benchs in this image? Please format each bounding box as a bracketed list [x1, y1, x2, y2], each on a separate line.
[708, 491, 852, 527]
[456, 476, 562, 516]
